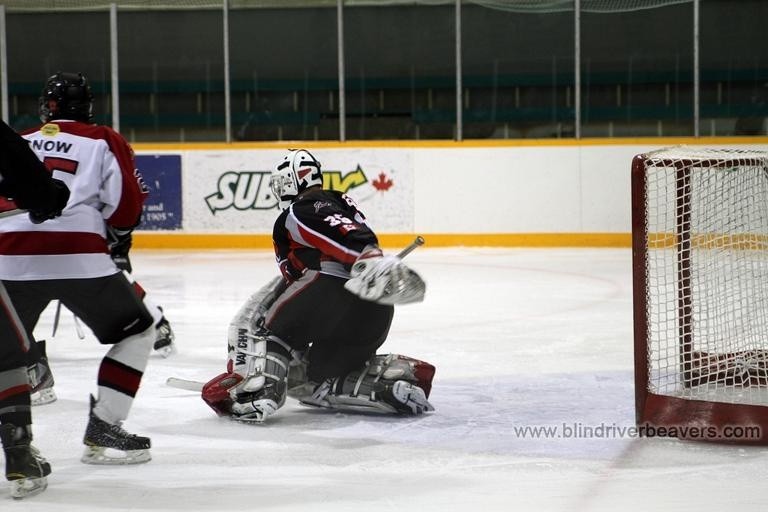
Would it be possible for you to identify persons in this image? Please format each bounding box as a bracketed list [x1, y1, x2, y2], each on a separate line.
[0, 120, 73, 479]
[0, 72, 159, 450]
[227, 148, 437, 421]
[23, 268, 174, 394]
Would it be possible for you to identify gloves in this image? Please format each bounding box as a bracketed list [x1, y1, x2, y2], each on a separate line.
[111, 230, 133, 274]
[30, 178, 70, 224]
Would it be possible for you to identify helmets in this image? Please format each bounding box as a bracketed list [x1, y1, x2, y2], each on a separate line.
[38, 72, 96, 124]
[270, 149, 323, 212]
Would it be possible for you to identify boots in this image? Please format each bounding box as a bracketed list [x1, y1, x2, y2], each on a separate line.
[154, 306, 171, 350]
[84, 394, 150, 450]
[27, 341, 54, 394]
[0, 423, 50, 480]
